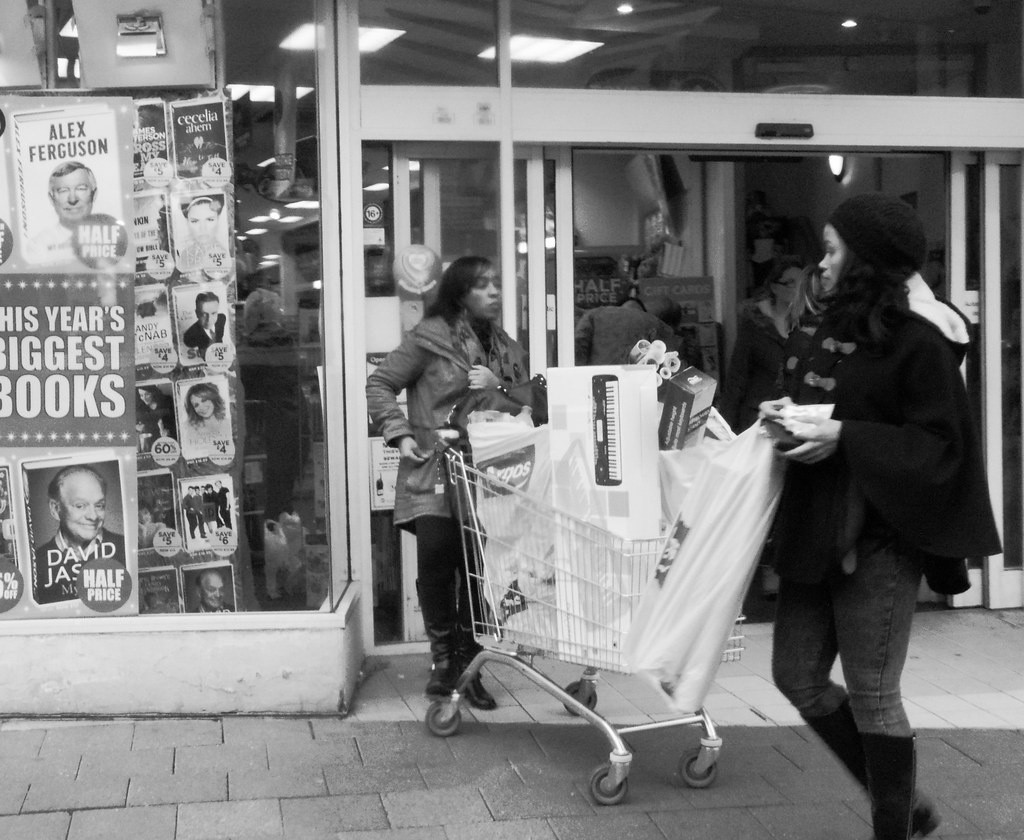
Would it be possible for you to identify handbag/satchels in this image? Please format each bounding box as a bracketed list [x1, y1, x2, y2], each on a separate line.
[447, 372, 548, 434]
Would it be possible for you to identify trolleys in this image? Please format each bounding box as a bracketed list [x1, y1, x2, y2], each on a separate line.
[423, 419, 730, 806]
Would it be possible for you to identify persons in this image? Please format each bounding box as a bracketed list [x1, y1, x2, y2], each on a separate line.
[31, 466, 125, 606]
[741, 192, 1002, 840]
[41, 162, 97, 254]
[130, 194, 240, 612]
[570, 296, 683, 365]
[237, 289, 312, 611]
[365, 253, 530, 711]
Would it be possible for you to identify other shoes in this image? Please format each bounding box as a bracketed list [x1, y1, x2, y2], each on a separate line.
[452, 657, 497, 710]
[761, 593, 779, 602]
[425, 653, 456, 696]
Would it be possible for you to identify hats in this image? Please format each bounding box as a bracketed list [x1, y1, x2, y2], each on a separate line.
[827, 191, 926, 285]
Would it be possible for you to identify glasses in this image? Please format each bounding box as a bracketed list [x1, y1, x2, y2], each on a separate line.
[774, 279, 796, 290]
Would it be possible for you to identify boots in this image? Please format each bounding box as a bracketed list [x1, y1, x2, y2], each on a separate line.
[858, 731, 917, 840]
[799, 693, 942, 836]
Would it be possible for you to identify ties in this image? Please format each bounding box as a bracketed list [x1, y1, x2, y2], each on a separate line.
[210, 329, 215, 342]
